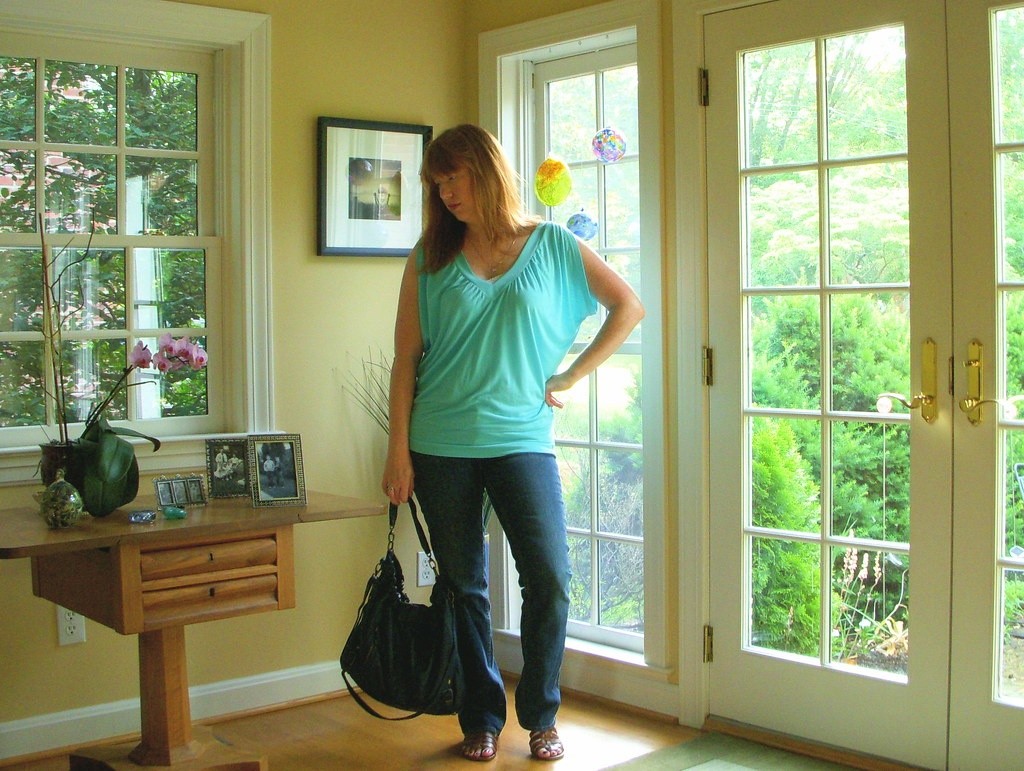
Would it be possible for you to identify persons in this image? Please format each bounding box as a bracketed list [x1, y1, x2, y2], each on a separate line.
[264, 454, 286, 488]
[382, 125, 646, 762]
[215, 448, 239, 481]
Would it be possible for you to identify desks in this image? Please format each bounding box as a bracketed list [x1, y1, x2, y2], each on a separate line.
[1, 489, 388, 771]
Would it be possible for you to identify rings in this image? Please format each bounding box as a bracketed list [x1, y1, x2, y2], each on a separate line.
[386, 482, 393, 490]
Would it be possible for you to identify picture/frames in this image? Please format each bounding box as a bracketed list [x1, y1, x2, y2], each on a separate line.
[204, 438, 250, 498]
[152, 473, 208, 510]
[247, 434, 307, 508]
[317, 116, 433, 257]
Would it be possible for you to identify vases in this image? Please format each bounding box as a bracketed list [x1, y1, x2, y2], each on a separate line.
[40, 442, 138, 518]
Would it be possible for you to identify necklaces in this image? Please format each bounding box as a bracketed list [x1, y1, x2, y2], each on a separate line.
[468, 231, 518, 274]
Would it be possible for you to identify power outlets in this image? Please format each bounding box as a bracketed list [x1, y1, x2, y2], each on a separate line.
[418, 552, 439, 587]
[56, 605, 86, 646]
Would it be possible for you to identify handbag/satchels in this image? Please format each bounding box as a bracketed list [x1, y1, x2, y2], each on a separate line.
[340, 495, 464, 721]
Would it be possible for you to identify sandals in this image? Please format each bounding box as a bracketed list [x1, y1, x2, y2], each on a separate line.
[461, 731, 498, 761]
[529, 727, 564, 761]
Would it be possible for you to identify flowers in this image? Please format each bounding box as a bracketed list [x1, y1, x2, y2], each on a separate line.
[9, 206, 208, 444]
